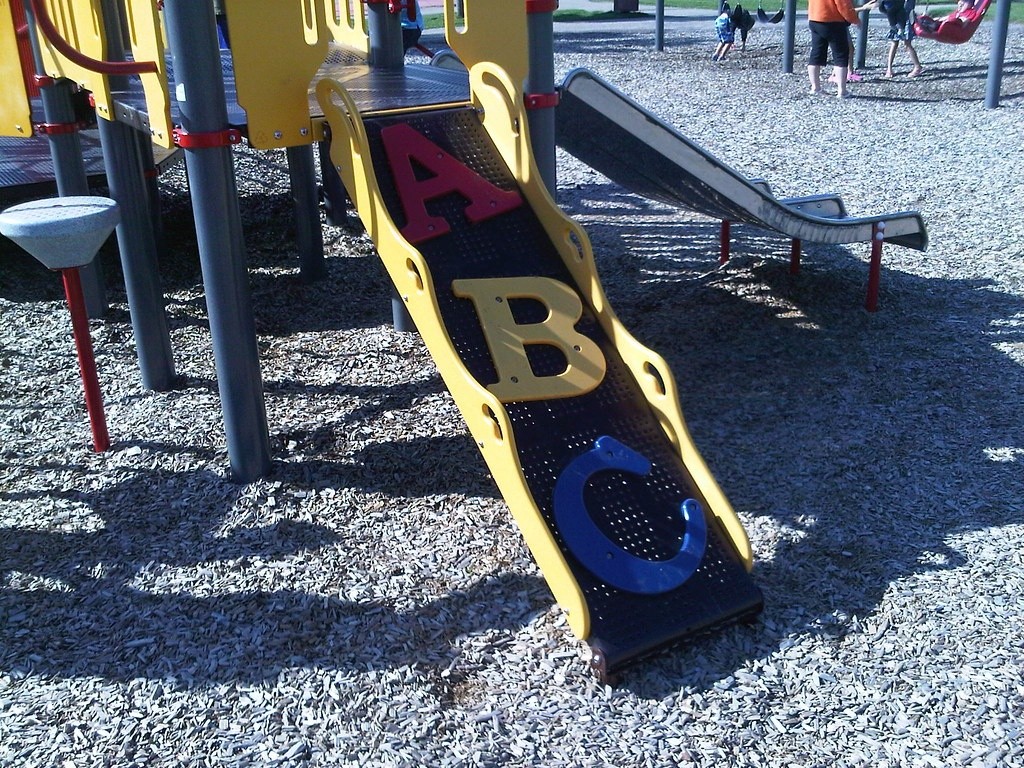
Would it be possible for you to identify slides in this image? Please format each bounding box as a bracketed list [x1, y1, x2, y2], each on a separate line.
[554, 67, 929, 252]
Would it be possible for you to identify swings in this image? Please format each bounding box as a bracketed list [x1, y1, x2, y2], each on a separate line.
[722, 0, 742, 20]
[912, 0, 990, 44]
[757, 0, 784, 24]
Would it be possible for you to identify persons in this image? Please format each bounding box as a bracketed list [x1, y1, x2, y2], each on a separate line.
[917, 0, 977, 36]
[807, 0, 923, 97]
[401, 0, 424, 55]
[711, 9, 750, 61]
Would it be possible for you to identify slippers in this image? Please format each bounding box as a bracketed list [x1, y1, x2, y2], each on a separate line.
[905, 68, 926, 79]
[885, 69, 892, 78]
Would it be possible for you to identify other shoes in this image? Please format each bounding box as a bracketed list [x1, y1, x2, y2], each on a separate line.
[716, 57, 729, 63]
[828, 75, 836, 82]
[909, 9, 918, 25]
[711, 55, 719, 61]
[847, 73, 863, 81]
[836, 91, 850, 98]
